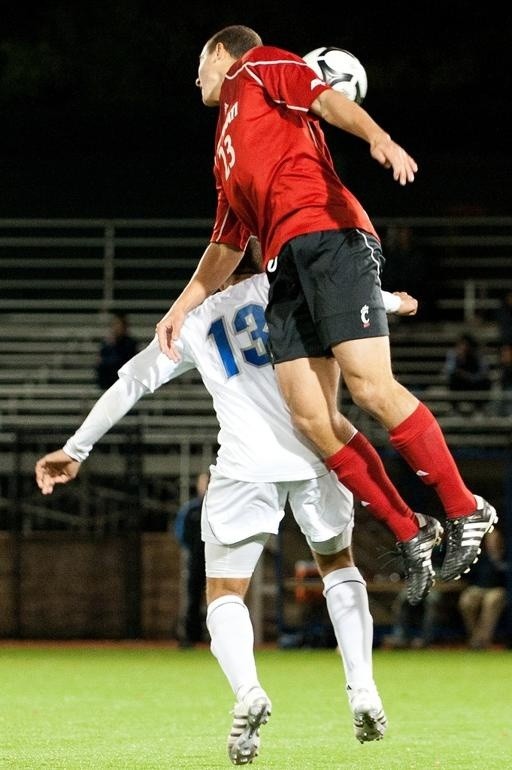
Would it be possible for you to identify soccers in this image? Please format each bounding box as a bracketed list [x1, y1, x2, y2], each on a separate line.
[304, 46, 370, 103]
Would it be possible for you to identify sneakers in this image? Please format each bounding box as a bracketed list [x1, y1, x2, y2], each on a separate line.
[394, 513, 445, 608]
[345, 680, 388, 744]
[436, 493, 499, 584]
[226, 680, 272, 766]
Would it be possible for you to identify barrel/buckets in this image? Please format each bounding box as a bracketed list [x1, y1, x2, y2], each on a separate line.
[295, 560, 325, 604]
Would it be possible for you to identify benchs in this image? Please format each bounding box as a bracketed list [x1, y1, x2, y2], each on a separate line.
[1, 314, 511, 474]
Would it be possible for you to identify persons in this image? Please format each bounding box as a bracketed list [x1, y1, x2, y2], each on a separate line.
[273, 220, 512, 651]
[172, 474, 212, 648]
[31, 226, 424, 769]
[90, 305, 147, 457]
[152, 22, 504, 611]
